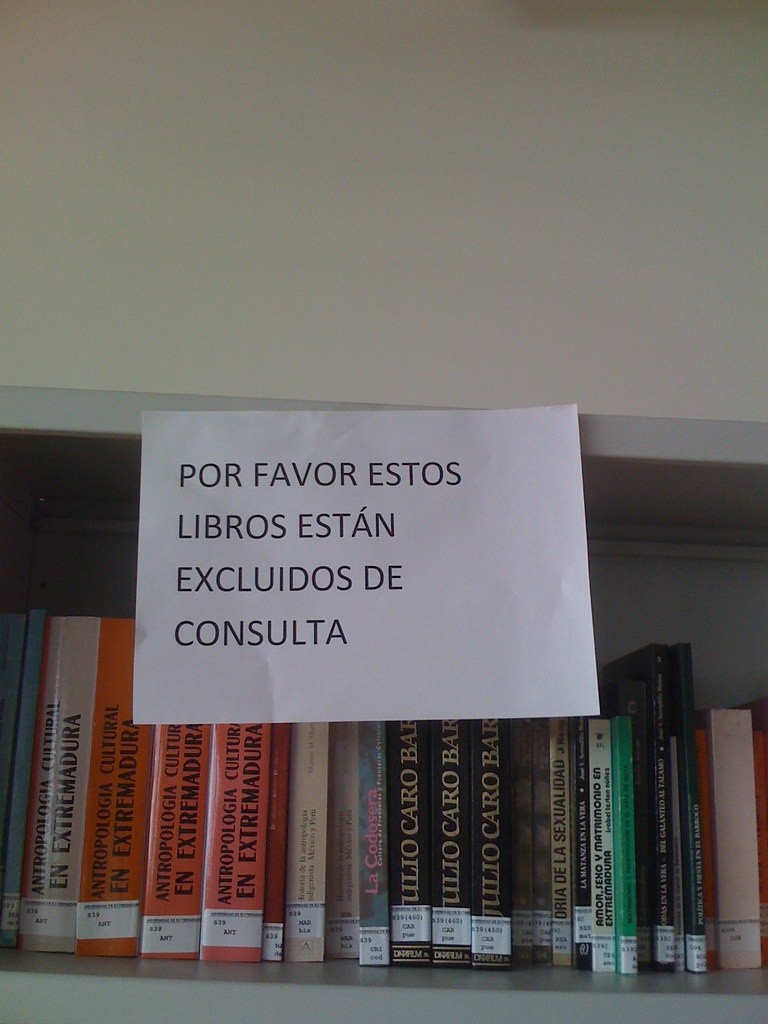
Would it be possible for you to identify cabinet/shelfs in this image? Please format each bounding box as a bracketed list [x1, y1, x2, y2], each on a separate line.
[0, 381, 768, 1024]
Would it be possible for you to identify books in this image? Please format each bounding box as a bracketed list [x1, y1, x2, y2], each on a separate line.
[0, 610, 768, 975]
